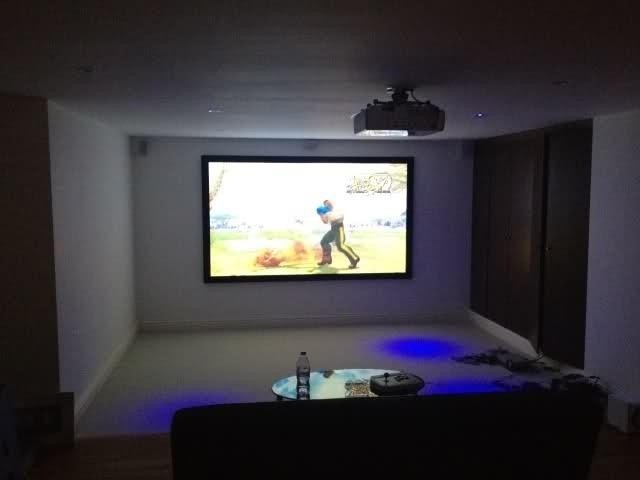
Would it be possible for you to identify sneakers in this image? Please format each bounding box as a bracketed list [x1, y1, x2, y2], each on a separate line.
[318, 258, 332, 266]
[348, 256, 361, 269]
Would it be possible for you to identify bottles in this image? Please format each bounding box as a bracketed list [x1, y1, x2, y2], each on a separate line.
[297, 351, 309, 387]
[298, 389, 308, 400]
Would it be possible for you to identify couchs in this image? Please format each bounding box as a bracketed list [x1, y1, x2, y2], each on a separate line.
[171, 385, 607, 480]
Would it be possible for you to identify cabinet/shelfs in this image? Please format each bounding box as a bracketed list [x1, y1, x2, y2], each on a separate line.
[470, 118, 593, 369]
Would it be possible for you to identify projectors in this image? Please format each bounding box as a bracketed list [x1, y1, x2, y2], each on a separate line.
[352, 101, 446, 137]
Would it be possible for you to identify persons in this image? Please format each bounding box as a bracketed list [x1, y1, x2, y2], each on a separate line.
[317, 198, 360, 269]
